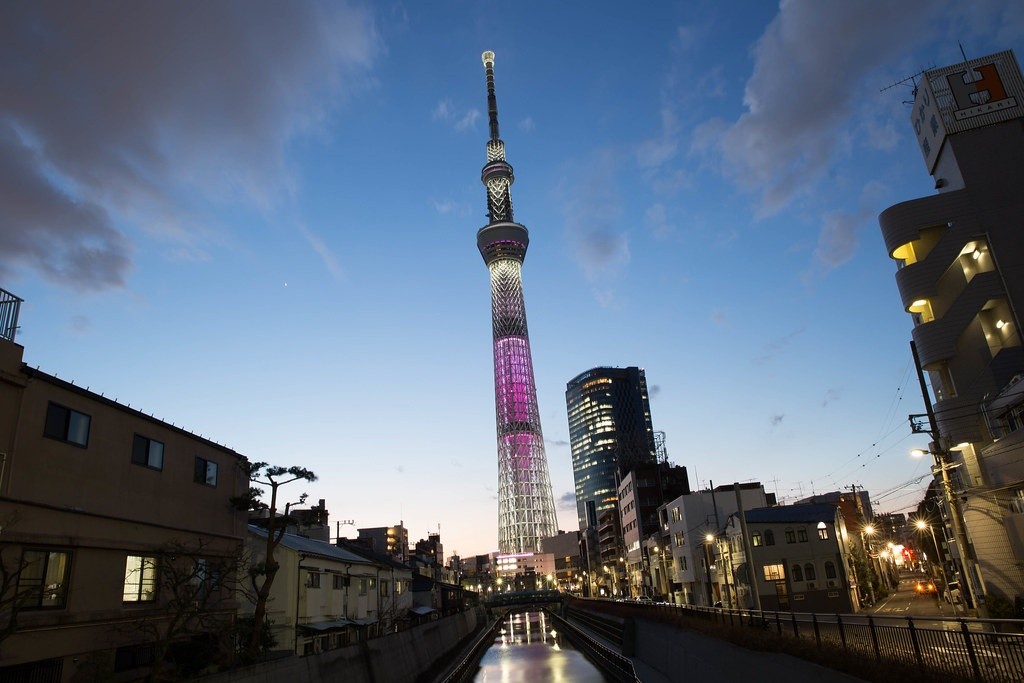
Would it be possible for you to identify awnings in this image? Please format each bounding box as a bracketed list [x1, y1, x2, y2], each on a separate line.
[301, 622, 345, 630]
[410, 605, 436, 615]
[352, 617, 379, 626]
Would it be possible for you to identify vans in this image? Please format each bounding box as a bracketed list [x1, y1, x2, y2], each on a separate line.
[944, 582, 962, 604]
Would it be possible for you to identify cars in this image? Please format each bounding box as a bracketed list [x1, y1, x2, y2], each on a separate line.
[917, 582, 933, 594]
[636, 596, 652, 603]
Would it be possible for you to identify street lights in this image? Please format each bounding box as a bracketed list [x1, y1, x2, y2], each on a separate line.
[908, 340, 981, 610]
[916, 520, 956, 615]
[863, 525, 877, 579]
[704, 480, 732, 607]
[653, 538, 672, 602]
